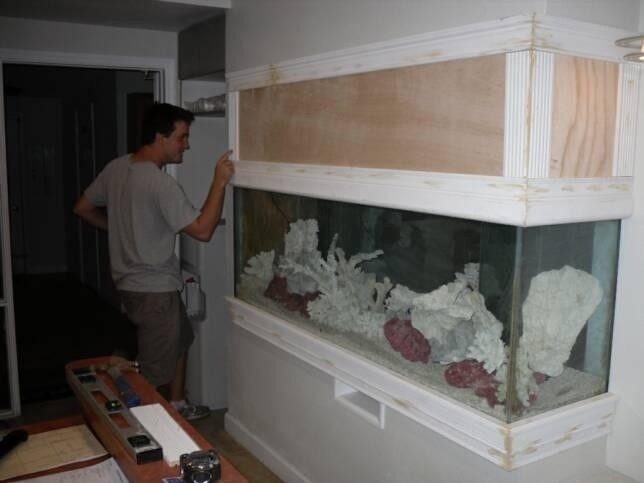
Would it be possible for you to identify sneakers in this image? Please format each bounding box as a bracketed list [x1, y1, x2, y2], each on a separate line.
[179, 270, 205, 322]
[175, 402, 211, 420]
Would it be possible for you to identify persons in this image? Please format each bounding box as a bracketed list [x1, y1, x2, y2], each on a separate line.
[72, 102, 234, 420]
